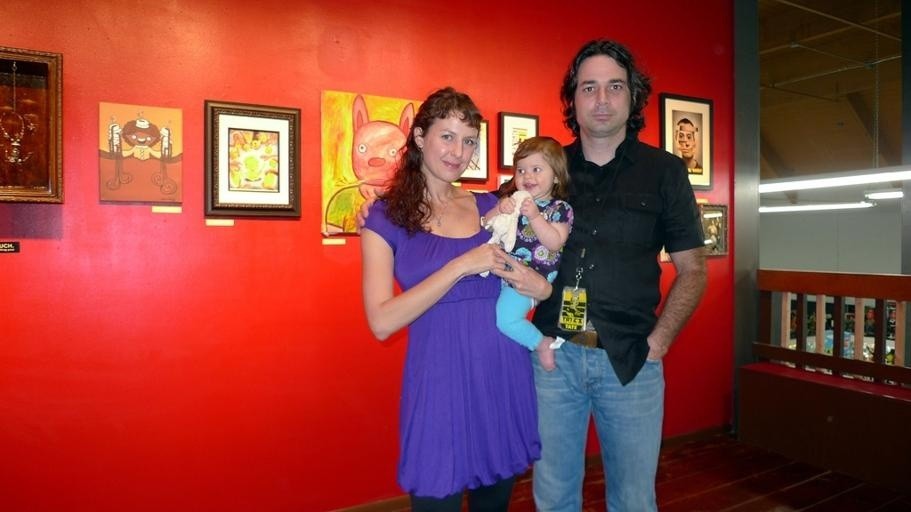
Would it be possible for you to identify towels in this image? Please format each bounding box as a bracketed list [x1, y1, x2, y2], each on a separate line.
[478, 190, 532, 278]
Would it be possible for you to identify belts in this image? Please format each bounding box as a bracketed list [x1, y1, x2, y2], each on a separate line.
[567, 329, 604, 350]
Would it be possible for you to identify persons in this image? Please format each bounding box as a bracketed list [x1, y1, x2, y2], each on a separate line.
[354, 39, 708, 512]
[675, 118, 702, 169]
[708, 220, 718, 248]
[480, 136, 575, 372]
[358, 87, 554, 512]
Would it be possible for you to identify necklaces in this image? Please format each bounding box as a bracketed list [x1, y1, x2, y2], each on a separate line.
[434, 191, 451, 227]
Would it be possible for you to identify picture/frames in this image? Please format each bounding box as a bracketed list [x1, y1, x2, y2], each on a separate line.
[659, 199, 708, 263]
[0, 47, 64, 204]
[659, 91, 713, 191]
[497, 173, 514, 191]
[500, 111, 540, 170]
[456, 119, 489, 182]
[205, 99, 302, 219]
[698, 204, 729, 257]
